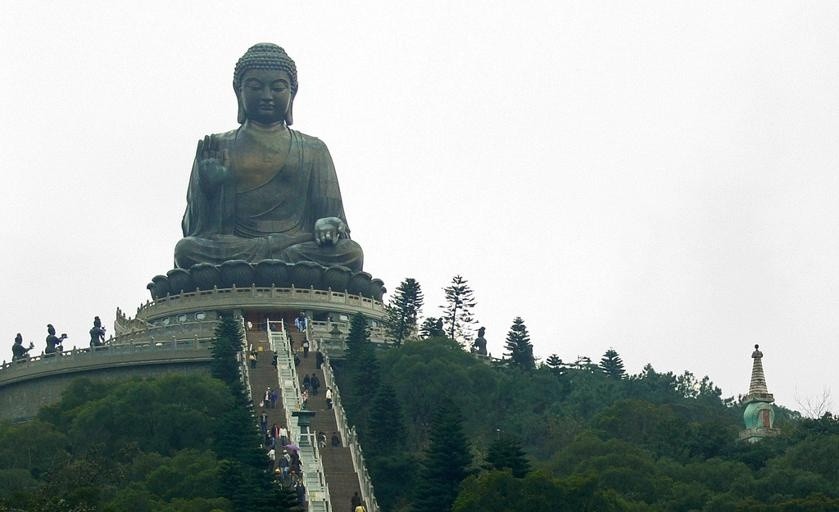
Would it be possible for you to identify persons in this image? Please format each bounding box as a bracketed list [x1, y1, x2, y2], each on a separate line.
[431, 318, 445, 339]
[332, 432, 340, 448]
[45, 324, 67, 353]
[12, 334, 34, 362]
[470, 326, 487, 355]
[255, 372, 333, 507]
[355, 502, 366, 512]
[90, 316, 104, 346]
[173, 41, 364, 274]
[244, 314, 339, 368]
[351, 491, 361, 512]
